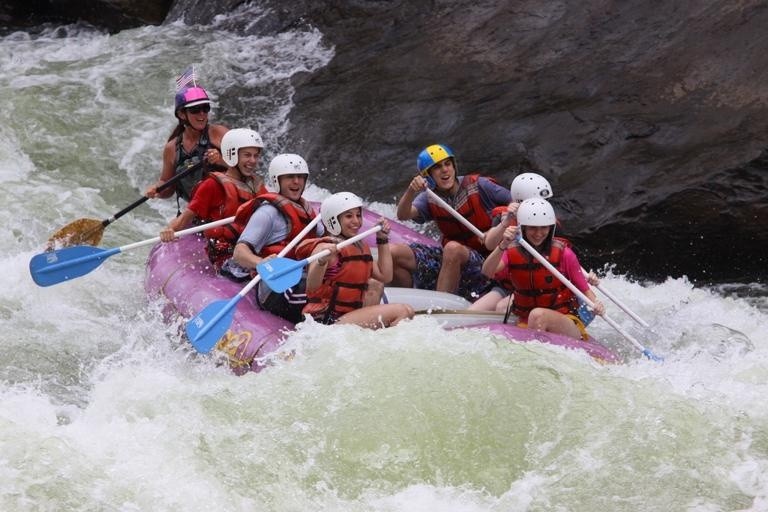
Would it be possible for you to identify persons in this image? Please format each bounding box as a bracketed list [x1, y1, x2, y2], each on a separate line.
[302, 191, 415, 331]
[233, 153, 385, 324]
[143, 87, 229, 205]
[468, 172, 600, 313]
[158, 127, 269, 283]
[481, 198, 606, 341]
[389, 144, 512, 303]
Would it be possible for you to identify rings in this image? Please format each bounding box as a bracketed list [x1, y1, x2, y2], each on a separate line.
[211, 152, 213, 156]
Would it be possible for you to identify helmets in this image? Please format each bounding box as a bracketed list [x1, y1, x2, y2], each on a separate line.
[268, 154, 310, 195]
[511, 173, 554, 203]
[321, 192, 363, 236]
[175, 85, 210, 119]
[416, 144, 458, 190]
[221, 128, 264, 167]
[517, 198, 557, 238]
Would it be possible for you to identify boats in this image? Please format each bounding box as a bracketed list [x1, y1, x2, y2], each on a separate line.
[143, 200, 622, 378]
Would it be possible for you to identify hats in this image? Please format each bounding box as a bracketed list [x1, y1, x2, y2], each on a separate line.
[184, 100, 214, 108]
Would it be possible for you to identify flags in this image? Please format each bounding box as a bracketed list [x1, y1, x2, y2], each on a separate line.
[175, 67, 194, 92]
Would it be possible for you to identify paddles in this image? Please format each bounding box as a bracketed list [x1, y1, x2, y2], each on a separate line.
[29, 217, 235, 287]
[186, 214, 320, 353]
[256, 225, 382, 294]
[514, 232, 667, 369]
[47, 158, 208, 251]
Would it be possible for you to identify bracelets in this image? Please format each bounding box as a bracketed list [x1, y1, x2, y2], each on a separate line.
[499, 245, 507, 251]
[376, 237, 388, 244]
[316, 258, 326, 266]
[501, 222, 510, 228]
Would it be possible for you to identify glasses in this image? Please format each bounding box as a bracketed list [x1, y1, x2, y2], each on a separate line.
[181, 104, 210, 114]
